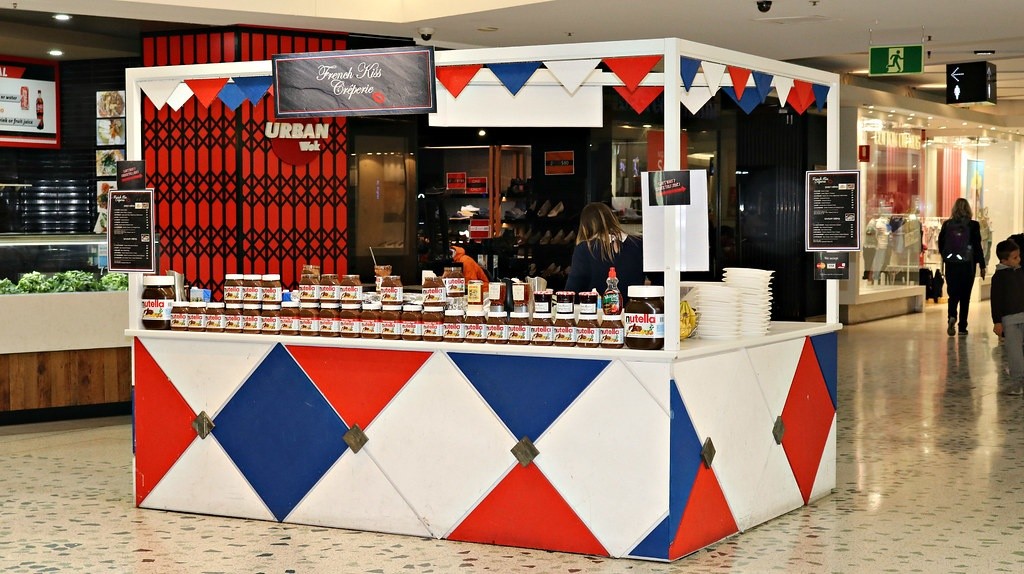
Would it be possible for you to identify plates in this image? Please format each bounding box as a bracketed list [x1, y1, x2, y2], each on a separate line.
[693, 267, 776, 340]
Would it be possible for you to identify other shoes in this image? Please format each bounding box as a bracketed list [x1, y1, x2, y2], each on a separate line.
[947, 316, 956, 336]
[958, 330, 968, 335]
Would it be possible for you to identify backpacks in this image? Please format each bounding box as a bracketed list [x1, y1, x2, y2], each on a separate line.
[944, 218, 970, 260]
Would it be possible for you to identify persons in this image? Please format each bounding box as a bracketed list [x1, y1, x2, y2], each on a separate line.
[433, 246, 491, 297]
[937, 198, 986, 336]
[990, 233, 1024, 395]
[564, 202, 664, 307]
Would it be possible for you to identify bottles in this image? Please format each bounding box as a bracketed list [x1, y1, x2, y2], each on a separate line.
[36, 90, 44, 129]
[133, 262, 667, 351]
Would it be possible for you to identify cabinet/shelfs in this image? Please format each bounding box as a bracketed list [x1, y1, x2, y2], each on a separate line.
[419, 144, 531, 279]
